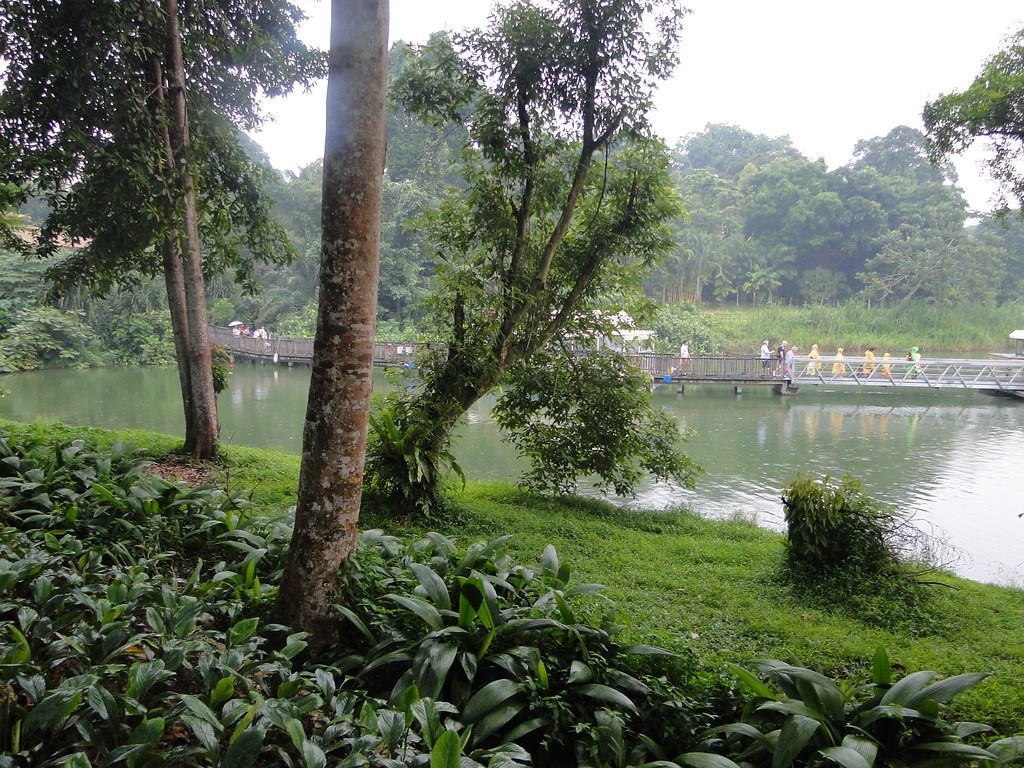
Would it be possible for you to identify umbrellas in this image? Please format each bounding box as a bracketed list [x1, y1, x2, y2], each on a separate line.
[229, 320, 243, 327]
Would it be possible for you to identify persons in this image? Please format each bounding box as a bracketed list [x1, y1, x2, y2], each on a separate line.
[233, 325, 273, 353]
[832, 348, 845, 379]
[679, 340, 691, 375]
[882, 353, 891, 378]
[761, 339, 775, 375]
[908, 347, 921, 379]
[863, 347, 875, 379]
[774, 341, 788, 377]
[784, 346, 798, 378]
[807, 344, 821, 378]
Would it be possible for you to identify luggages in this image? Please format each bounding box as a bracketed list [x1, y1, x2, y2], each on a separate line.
[672, 365, 679, 377]
